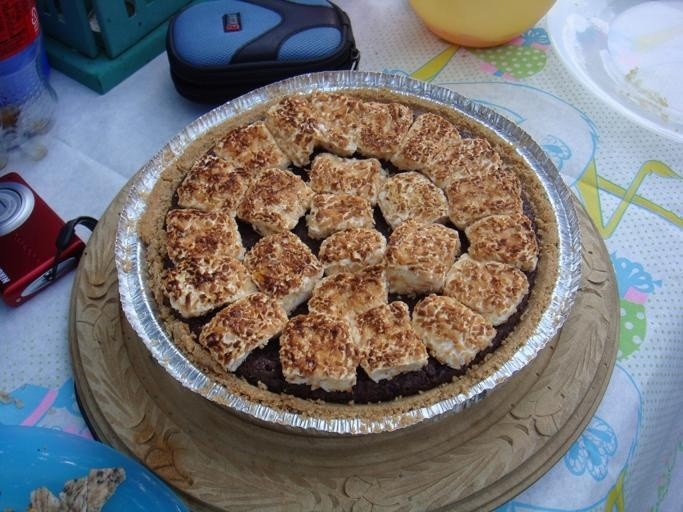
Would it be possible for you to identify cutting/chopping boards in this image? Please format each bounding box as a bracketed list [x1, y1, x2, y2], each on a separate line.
[66, 162, 622, 512]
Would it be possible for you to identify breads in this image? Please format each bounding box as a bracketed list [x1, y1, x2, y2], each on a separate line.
[138, 87, 558, 421]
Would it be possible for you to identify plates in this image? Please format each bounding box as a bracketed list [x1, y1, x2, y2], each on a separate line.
[0, 424, 190, 512]
[546, 0, 683, 144]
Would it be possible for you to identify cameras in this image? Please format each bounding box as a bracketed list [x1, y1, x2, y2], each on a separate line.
[0, 172, 86, 307]
